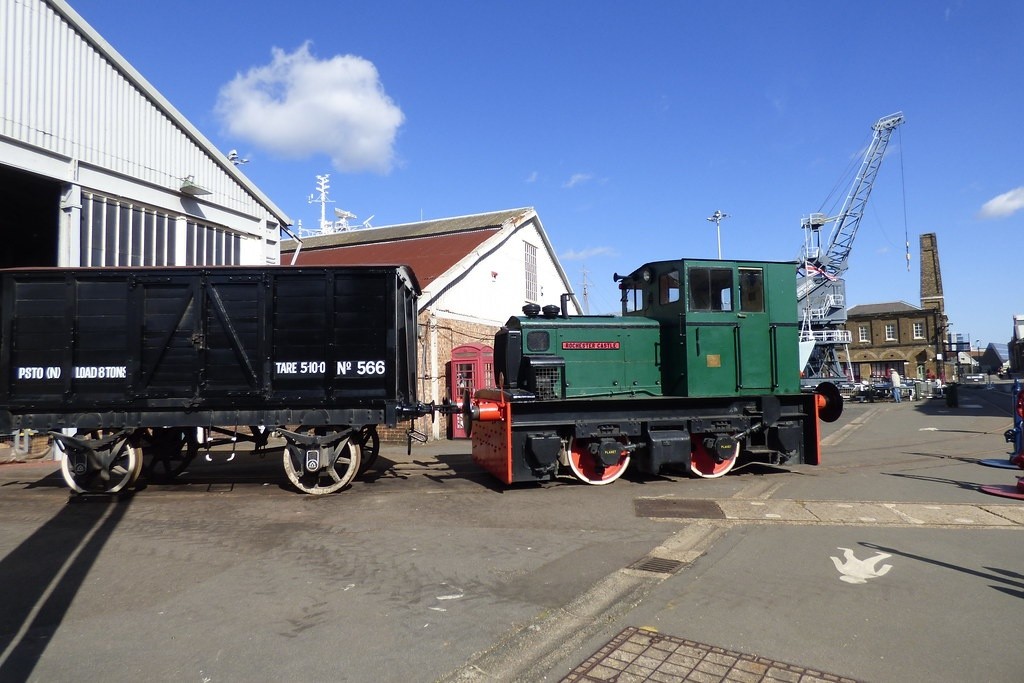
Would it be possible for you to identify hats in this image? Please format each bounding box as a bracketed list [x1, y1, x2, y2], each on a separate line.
[890, 368, 894, 370]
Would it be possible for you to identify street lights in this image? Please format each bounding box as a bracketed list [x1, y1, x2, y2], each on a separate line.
[707, 209, 729, 259]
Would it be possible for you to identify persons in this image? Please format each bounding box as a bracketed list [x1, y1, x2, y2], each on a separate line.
[889, 367, 901, 403]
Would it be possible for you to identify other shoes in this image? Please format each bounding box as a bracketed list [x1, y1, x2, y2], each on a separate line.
[894, 400, 901, 403]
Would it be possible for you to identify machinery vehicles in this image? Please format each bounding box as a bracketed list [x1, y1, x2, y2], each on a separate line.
[0, 249, 843, 499]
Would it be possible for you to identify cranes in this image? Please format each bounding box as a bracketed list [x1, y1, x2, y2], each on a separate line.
[792, 109, 911, 401]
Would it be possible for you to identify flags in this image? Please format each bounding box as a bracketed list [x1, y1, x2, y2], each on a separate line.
[806, 261, 837, 282]
